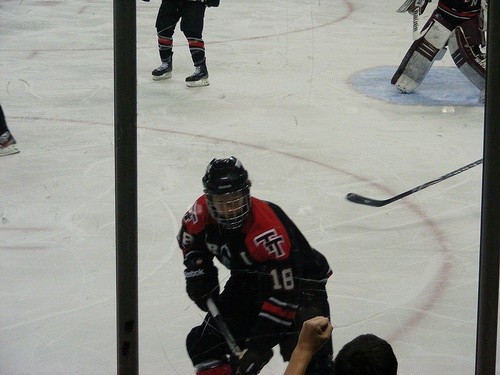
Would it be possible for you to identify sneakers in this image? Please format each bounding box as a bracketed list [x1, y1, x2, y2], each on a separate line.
[0, 131, 20, 156]
[186, 64, 210, 87]
[152, 58, 173, 81]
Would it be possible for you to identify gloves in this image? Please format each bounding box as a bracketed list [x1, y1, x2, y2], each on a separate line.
[229, 337, 273, 375]
[183, 259, 220, 312]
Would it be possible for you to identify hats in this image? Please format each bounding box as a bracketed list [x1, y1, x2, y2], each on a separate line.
[335, 334, 398, 375]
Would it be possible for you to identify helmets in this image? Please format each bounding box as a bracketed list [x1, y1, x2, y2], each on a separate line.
[202, 156, 252, 230]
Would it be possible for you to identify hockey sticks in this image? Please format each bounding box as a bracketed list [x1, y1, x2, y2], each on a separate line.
[345, 157, 483, 208]
[206, 299, 244, 362]
[411, 6, 435, 41]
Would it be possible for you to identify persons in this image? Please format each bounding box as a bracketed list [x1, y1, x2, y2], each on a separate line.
[391, 0, 488, 103]
[176, 155, 335, 375]
[0, 104, 16, 149]
[282, 315, 398, 375]
[143, 0, 220, 81]
